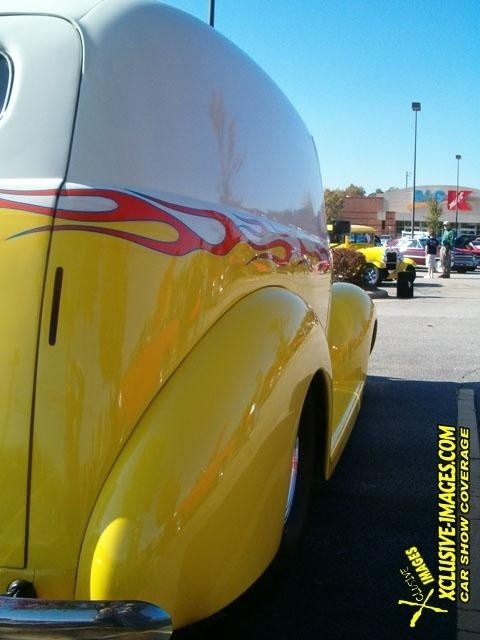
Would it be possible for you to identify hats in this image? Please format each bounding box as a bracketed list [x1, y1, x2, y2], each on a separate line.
[442, 221, 451, 226]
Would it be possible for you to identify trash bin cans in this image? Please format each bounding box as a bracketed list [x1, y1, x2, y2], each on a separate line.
[397, 271, 414, 299]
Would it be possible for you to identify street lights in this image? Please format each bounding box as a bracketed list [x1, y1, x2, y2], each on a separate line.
[455, 154, 461, 238]
[411, 101, 421, 238]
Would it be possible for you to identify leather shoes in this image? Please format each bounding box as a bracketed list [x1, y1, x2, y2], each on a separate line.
[438, 275, 450, 278]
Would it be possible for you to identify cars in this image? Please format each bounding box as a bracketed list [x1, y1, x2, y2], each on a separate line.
[326, 224, 417, 290]
[0, 0, 378, 640]
[380, 235, 480, 273]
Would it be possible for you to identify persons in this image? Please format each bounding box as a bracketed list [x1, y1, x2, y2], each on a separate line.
[438, 220, 455, 278]
[424, 229, 441, 279]
